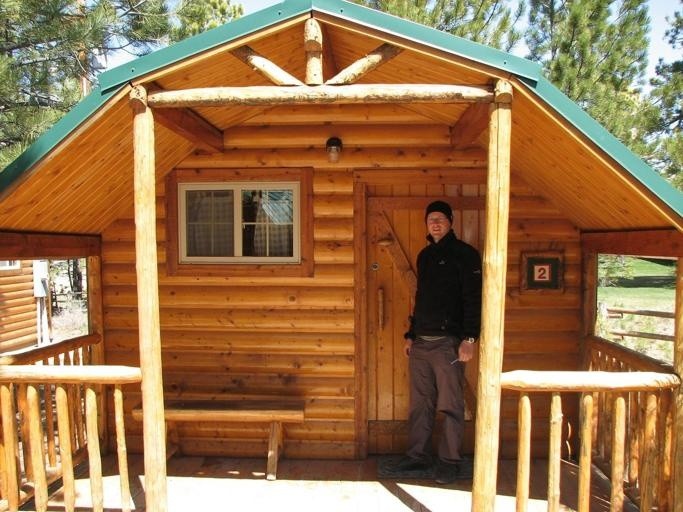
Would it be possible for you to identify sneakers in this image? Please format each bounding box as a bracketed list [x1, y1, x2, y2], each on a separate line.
[377, 455, 425, 479]
[435, 463, 458, 484]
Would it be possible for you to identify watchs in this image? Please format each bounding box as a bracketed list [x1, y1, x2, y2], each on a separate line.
[464, 336, 476, 343]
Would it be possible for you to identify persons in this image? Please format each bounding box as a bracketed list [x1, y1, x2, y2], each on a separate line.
[378, 201, 482, 485]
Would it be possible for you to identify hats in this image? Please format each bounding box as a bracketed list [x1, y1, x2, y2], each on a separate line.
[425, 201, 453, 225]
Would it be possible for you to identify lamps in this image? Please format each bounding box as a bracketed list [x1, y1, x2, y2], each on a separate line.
[325, 137, 343, 164]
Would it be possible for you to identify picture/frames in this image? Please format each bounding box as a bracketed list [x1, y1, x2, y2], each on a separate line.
[520, 252, 564, 295]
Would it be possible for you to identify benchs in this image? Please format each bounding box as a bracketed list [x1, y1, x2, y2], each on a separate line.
[130, 401, 305, 479]
